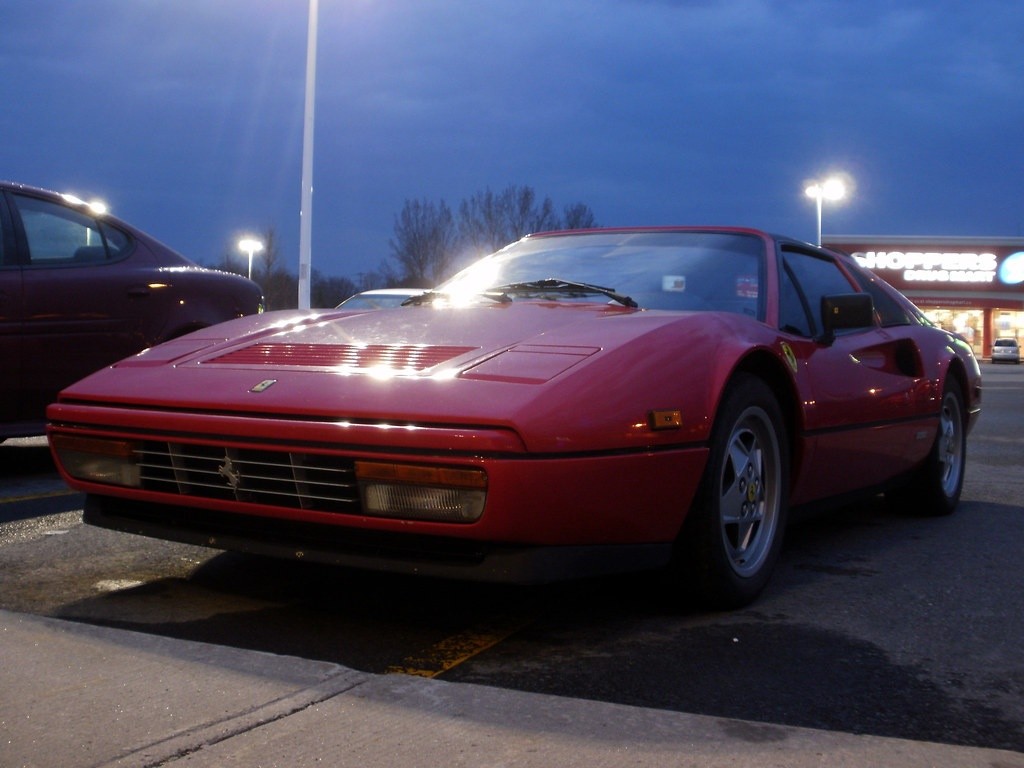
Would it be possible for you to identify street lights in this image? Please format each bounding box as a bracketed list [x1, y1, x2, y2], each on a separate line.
[805, 178, 840, 248]
[238, 237, 264, 281]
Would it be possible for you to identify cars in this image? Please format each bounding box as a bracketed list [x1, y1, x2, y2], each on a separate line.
[0, 178, 265, 458]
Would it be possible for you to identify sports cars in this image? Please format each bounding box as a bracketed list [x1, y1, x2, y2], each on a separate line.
[39, 222, 984, 614]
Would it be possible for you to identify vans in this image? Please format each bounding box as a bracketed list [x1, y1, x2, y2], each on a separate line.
[990, 337, 1021, 364]
